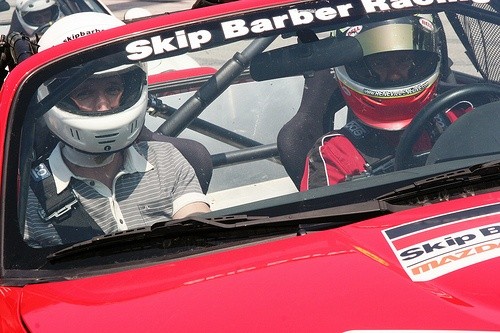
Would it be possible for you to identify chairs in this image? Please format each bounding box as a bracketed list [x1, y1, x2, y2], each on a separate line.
[277, 66, 490, 193]
[31, 125, 214, 196]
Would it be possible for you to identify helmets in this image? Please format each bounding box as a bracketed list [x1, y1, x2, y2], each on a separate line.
[334, 11, 443, 129]
[16, 0, 62, 35]
[33, 12, 149, 154]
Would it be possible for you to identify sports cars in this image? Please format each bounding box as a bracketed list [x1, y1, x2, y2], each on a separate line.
[1, 0, 500, 330]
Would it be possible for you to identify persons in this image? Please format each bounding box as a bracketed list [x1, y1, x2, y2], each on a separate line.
[24, 12, 211, 248]
[16, 0, 64, 36]
[299, 14, 476, 192]
